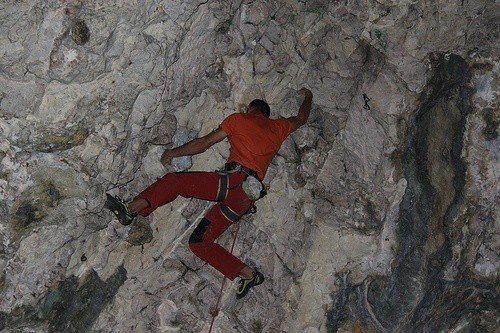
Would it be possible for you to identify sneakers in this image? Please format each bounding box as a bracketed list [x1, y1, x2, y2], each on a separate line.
[235, 267, 265, 300]
[106, 192, 137, 225]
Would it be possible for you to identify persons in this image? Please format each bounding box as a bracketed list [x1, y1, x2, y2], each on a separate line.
[102, 84, 313, 301]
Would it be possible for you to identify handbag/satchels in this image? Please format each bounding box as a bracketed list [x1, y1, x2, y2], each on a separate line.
[242, 175, 263, 200]
